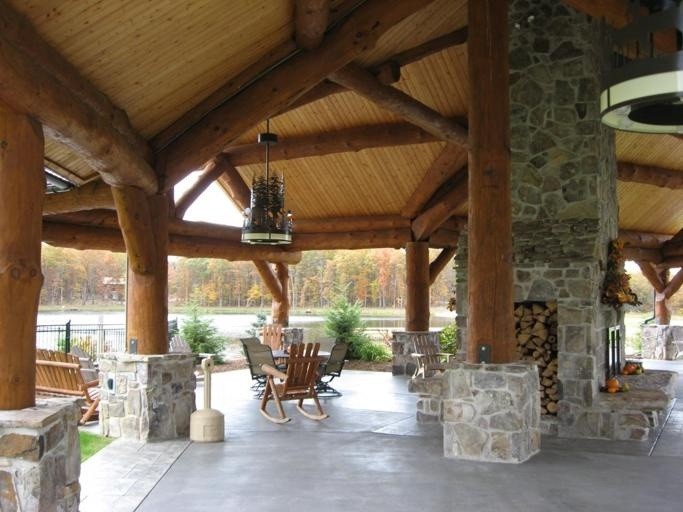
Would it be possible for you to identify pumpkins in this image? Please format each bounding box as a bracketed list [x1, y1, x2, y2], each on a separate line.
[607, 378, 619, 387]
[626, 363, 637, 372]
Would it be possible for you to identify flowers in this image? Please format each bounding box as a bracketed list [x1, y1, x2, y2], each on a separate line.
[602, 236, 643, 309]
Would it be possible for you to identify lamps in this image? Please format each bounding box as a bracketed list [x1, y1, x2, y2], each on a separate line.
[599, 1, 683, 135]
[239, 118, 294, 245]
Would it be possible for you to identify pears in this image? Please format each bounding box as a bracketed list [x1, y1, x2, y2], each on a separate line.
[622, 381, 630, 391]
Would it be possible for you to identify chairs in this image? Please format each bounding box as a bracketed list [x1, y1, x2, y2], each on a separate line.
[409, 334, 454, 380]
[169, 335, 216, 378]
[35, 345, 103, 425]
[239, 322, 352, 425]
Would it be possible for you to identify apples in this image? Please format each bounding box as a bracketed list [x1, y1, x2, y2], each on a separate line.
[636, 368, 642, 375]
